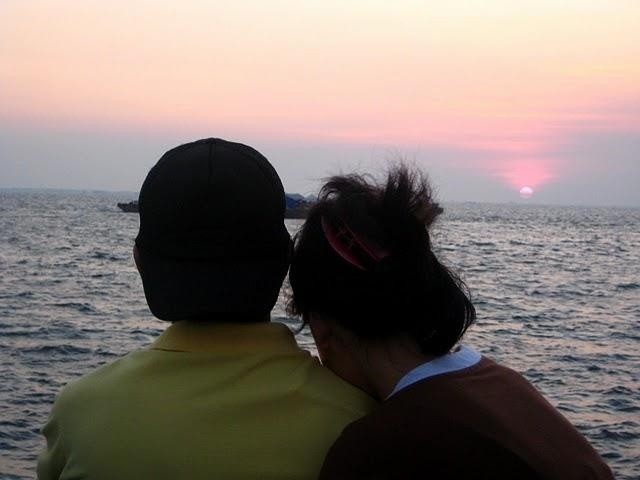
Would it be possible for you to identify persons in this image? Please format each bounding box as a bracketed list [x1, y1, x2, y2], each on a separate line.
[284, 160, 615, 479]
[36, 137, 385, 479]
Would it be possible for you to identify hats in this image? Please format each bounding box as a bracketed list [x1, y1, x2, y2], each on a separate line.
[135, 138, 294, 323]
